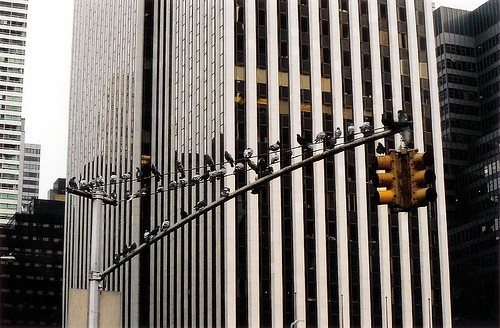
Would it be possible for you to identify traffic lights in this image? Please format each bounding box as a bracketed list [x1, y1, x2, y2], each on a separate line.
[370, 148, 438, 211]
